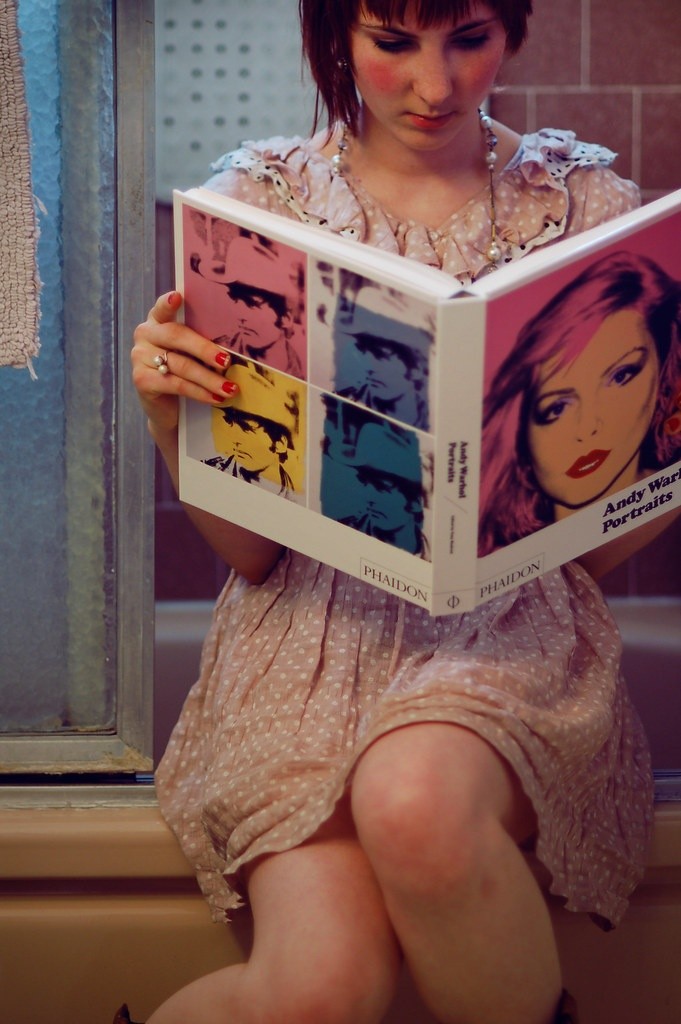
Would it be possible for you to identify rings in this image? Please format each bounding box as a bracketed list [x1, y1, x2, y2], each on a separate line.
[153, 350, 173, 375]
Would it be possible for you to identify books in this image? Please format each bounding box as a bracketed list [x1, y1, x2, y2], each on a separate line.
[174, 189, 680, 618]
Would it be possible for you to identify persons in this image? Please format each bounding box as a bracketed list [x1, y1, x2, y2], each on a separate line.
[477, 252, 681, 557]
[198, 238, 436, 565]
[132, 0, 681, 1024]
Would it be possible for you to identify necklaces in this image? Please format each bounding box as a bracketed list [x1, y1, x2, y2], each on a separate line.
[332, 108, 501, 274]
